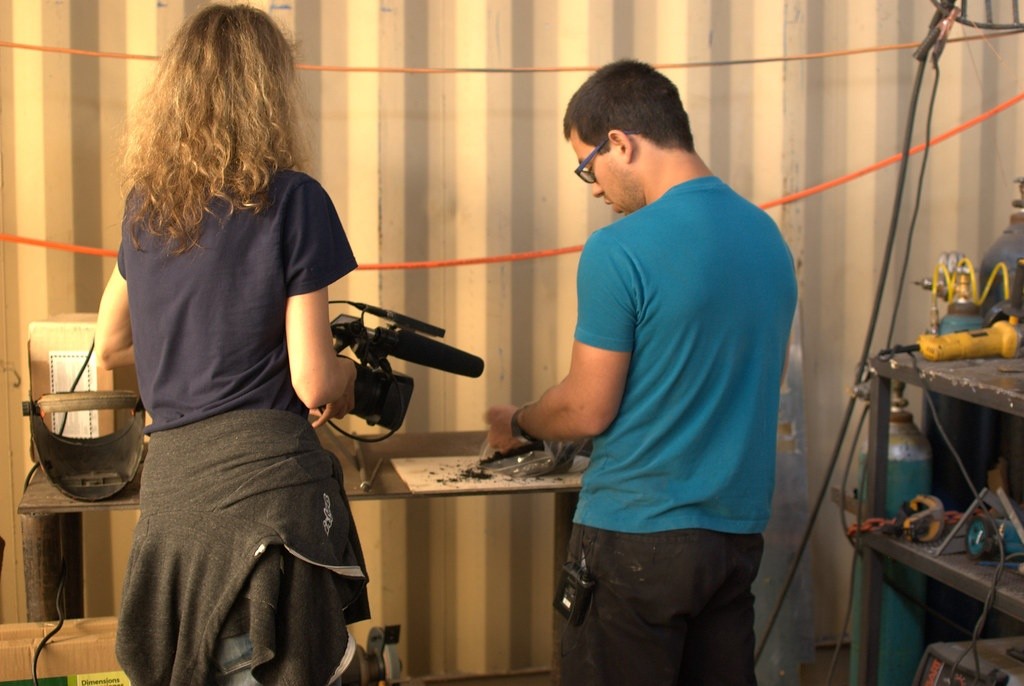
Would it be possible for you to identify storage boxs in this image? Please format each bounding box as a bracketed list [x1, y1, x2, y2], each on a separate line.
[25, 313, 138, 438]
[0, 616, 131, 686]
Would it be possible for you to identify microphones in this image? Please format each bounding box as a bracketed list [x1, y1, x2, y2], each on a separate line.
[372, 327, 485, 379]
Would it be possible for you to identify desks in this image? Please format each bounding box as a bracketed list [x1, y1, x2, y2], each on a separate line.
[16, 431, 589, 686]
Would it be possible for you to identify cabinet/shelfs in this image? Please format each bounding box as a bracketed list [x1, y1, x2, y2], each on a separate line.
[853, 349, 1024, 686]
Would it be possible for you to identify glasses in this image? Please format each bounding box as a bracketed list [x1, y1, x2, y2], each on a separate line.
[574, 128, 641, 183]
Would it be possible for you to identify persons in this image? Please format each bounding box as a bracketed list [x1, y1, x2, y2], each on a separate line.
[486, 59, 798, 686]
[95, 4, 372, 686]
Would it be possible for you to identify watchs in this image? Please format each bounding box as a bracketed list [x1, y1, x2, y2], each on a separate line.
[511, 408, 538, 444]
